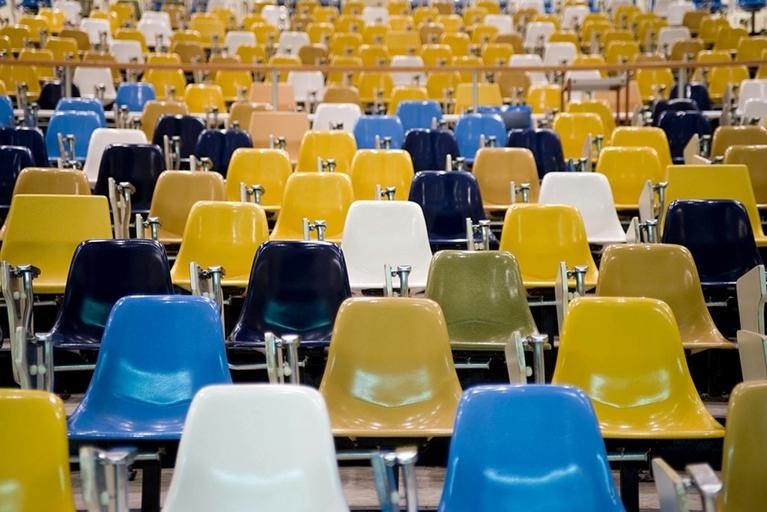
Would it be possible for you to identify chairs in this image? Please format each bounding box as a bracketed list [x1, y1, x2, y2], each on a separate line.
[0, 0, 767, 511]
